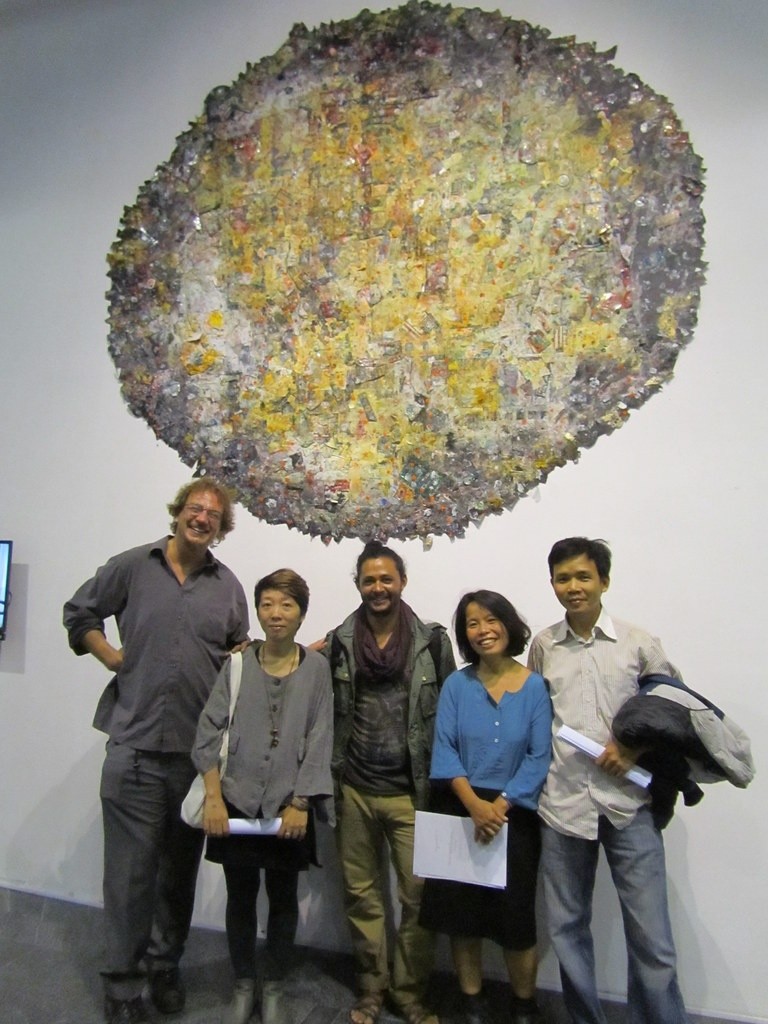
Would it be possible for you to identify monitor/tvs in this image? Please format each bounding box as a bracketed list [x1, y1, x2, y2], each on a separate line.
[0, 540, 12, 631]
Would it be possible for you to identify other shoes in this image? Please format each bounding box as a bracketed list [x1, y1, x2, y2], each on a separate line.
[262, 976, 292, 1024]
[221, 978, 258, 1024]
[146, 970, 184, 1013]
[104, 996, 147, 1024]
[511, 994, 540, 1024]
[461, 986, 488, 1024]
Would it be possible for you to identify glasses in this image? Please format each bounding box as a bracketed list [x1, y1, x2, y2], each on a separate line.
[183, 505, 223, 523]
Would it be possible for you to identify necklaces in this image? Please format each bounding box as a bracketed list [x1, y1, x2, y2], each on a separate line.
[262, 641, 297, 748]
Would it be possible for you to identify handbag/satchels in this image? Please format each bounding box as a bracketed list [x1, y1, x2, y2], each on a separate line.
[179, 652, 243, 829]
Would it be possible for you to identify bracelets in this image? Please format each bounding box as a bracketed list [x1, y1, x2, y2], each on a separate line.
[500, 791, 513, 808]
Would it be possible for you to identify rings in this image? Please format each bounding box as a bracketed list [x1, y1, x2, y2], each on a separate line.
[285, 832, 290, 839]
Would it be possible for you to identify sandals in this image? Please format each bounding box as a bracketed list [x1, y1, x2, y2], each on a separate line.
[348, 989, 388, 1024]
[388, 1002, 439, 1024]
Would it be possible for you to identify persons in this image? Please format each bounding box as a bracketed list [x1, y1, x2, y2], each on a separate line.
[191, 568, 333, 1024]
[526, 537, 686, 1024]
[417, 590, 554, 1024]
[226, 541, 458, 1024]
[63, 478, 329, 1024]
